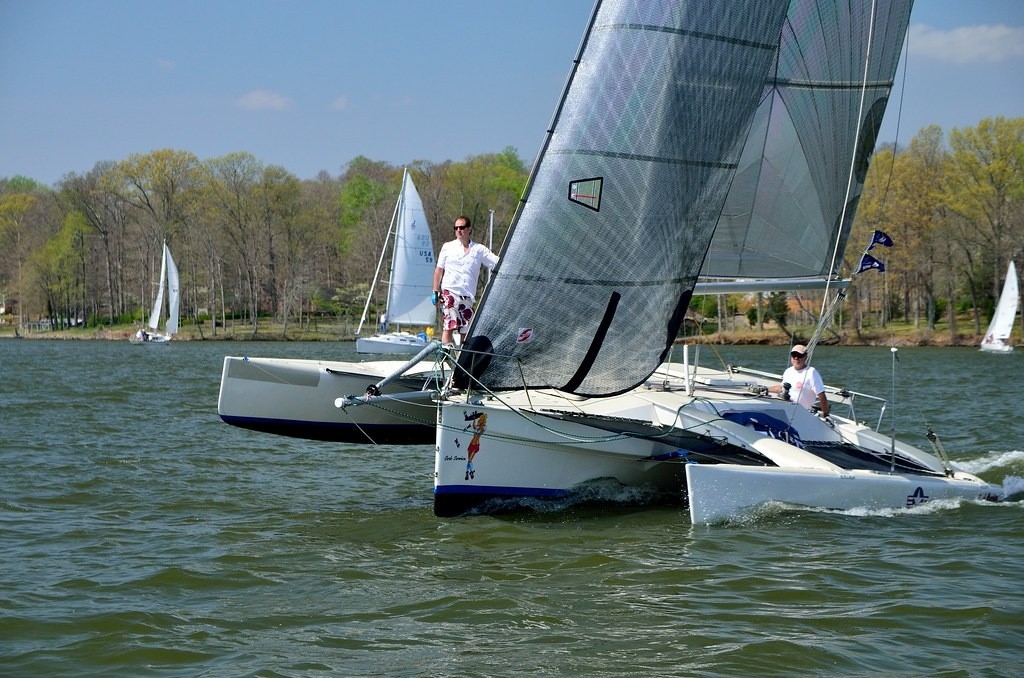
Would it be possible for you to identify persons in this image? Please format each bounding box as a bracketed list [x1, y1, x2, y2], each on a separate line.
[431, 216, 501, 344]
[747, 345, 829, 418]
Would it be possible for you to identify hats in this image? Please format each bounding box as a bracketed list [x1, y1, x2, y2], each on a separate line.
[791, 344, 807, 354]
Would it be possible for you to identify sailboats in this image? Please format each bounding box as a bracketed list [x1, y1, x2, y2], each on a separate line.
[980, 261, 1020, 352]
[212, 0, 1009, 524]
[128, 238, 181, 345]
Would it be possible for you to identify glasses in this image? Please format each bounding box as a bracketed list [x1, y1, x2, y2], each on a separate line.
[453, 224, 468, 230]
[792, 354, 805, 358]
